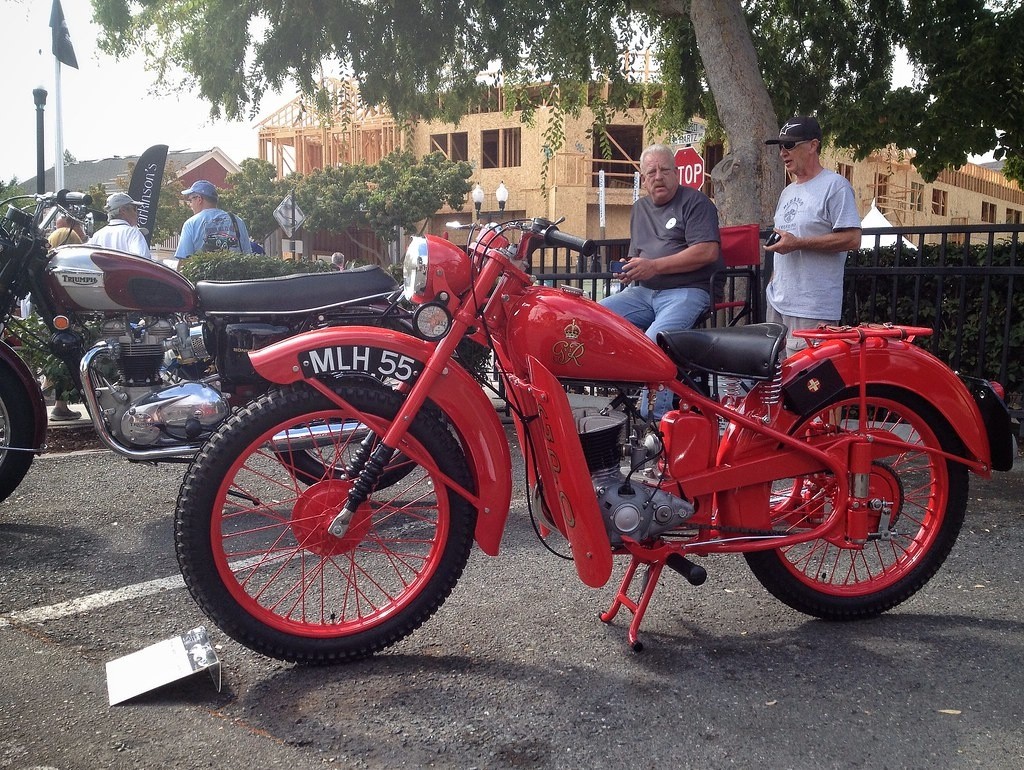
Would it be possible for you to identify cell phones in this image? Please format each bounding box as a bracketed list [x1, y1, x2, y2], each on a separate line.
[610, 261, 632, 273]
[766, 233, 781, 246]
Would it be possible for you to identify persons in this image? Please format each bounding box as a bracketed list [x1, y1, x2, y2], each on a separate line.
[88, 193, 151, 258]
[597, 144, 727, 421]
[765, 118, 863, 426]
[176, 180, 251, 272]
[41, 217, 89, 421]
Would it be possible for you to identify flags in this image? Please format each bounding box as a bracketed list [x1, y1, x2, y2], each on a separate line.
[49, 0, 79, 70]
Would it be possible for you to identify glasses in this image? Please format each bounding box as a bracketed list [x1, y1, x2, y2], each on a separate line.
[125, 206, 137, 212]
[779, 139, 821, 151]
[188, 196, 198, 203]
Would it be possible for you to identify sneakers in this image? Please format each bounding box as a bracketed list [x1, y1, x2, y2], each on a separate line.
[50, 405, 81, 421]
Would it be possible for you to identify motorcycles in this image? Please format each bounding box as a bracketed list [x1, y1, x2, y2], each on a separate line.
[175, 214, 1018, 670]
[0, 190, 424, 498]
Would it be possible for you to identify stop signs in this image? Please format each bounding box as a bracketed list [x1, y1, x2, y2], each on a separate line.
[674, 146, 704, 191]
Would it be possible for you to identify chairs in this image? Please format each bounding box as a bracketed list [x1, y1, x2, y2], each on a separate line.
[709, 223, 761, 326]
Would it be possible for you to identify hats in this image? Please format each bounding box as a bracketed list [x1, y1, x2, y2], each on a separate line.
[181, 179, 218, 199]
[764, 117, 823, 145]
[105, 192, 144, 213]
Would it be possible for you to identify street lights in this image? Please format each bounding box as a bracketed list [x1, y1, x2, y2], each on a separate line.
[32, 84, 50, 195]
